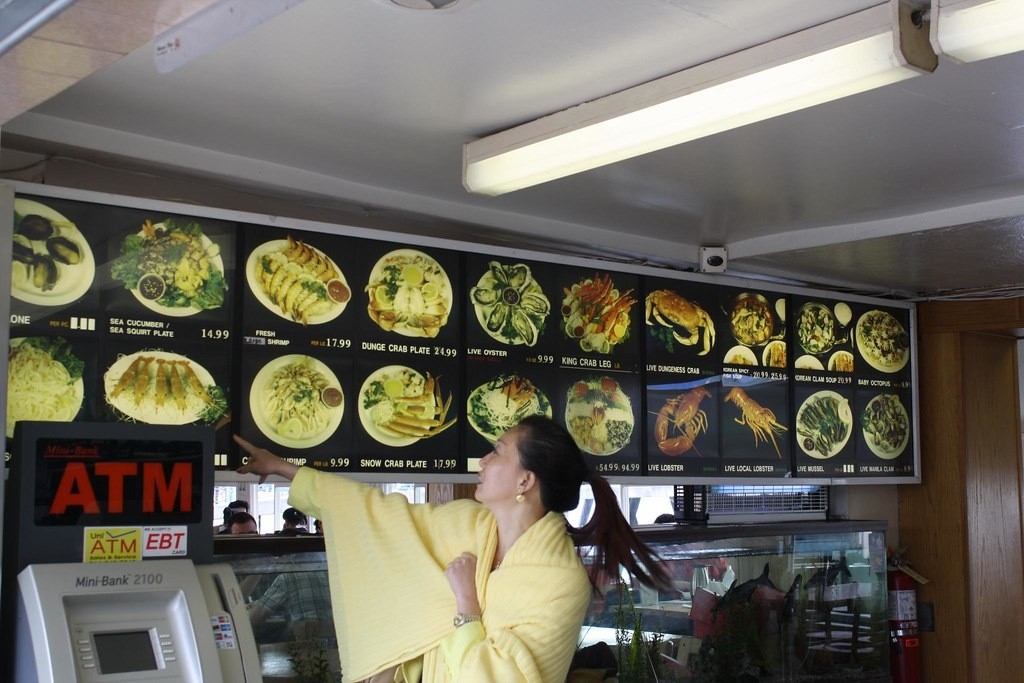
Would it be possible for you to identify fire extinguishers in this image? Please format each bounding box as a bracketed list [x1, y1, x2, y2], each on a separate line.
[886, 546, 927, 683]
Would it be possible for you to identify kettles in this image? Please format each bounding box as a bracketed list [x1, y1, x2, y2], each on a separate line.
[690, 563, 711, 607]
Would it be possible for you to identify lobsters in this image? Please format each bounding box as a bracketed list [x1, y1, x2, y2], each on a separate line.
[654, 383, 714, 458]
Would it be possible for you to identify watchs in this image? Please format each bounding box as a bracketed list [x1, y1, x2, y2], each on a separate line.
[454, 613, 481, 627]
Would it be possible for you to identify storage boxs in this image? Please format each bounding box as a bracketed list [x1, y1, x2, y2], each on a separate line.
[215, 519, 891, 683]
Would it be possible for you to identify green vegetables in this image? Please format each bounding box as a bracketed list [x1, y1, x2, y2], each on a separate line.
[468, 380, 551, 436]
[379, 264, 405, 302]
[363, 380, 385, 410]
[109, 216, 229, 311]
[196, 384, 229, 423]
[18, 336, 85, 384]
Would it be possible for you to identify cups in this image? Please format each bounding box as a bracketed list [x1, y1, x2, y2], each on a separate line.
[682, 591, 691, 601]
[833, 302, 853, 329]
[640, 578, 659, 606]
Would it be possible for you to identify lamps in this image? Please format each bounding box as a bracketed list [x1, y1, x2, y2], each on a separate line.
[929, 0, 1024, 65]
[461, 0, 938, 197]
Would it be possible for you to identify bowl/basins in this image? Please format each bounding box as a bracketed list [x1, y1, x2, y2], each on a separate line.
[871, 399, 882, 413]
[803, 437, 815, 452]
[898, 332, 910, 349]
[327, 278, 352, 306]
[775, 299, 786, 325]
[136, 273, 166, 302]
[466, 378, 552, 449]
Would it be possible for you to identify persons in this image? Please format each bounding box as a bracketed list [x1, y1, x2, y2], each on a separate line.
[231, 414, 682, 683]
[213, 499, 336, 640]
[575, 512, 727, 614]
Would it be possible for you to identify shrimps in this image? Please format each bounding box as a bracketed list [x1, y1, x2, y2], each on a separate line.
[110, 356, 208, 409]
[253, 232, 340, 327]
[723, 387, 789, 460]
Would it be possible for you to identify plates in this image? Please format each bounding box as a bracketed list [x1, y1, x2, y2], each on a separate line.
[796, 390, 853, 460]
[762, 340, 786, 368]
[245, 240, 352, 325]
[368, 249, 453, 337]
[120, 220, 224, 317]
[674, 600, 692, 603]
[723, 345, 758, 366]
[321, 386, 344, 409]
[249, 354, 344, 449]
[9, 198, 95, 306]
[564, 379, 635, 456]
[5, 336, 84, 440]
[794, 355, 826, 370]
[473, 265, 546, 345]
[862, 392, 909, 460]
[827, 350, 854, 372]
[855, 310, 910, 373]
[358, 365, 436, 447]
[104, 350, 217, 425]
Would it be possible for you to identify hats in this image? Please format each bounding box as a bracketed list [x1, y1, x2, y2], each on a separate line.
[282, 508, 303, 523]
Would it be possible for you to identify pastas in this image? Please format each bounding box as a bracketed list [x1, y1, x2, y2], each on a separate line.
[8, 341, 77, 434]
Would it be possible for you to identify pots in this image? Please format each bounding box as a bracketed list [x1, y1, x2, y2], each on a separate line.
[792, 300, 849, 355]
[721, 291, 786, 348]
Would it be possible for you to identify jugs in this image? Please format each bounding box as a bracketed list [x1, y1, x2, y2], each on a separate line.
[705, 578, 723, 597]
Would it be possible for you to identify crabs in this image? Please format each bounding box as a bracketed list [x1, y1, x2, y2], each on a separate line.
[643, 288, 715, 354]
[571, 270, 639, 342]
[369, 370, 459, 440]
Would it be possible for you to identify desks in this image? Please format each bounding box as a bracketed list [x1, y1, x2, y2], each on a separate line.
[610, 600, 693, 635]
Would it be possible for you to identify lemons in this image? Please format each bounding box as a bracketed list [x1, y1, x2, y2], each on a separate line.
[421, 283, 438, 300]
[614, 324, 625, 338]
[375, 286, 392, 306]
[403, 265, 424, 287]
